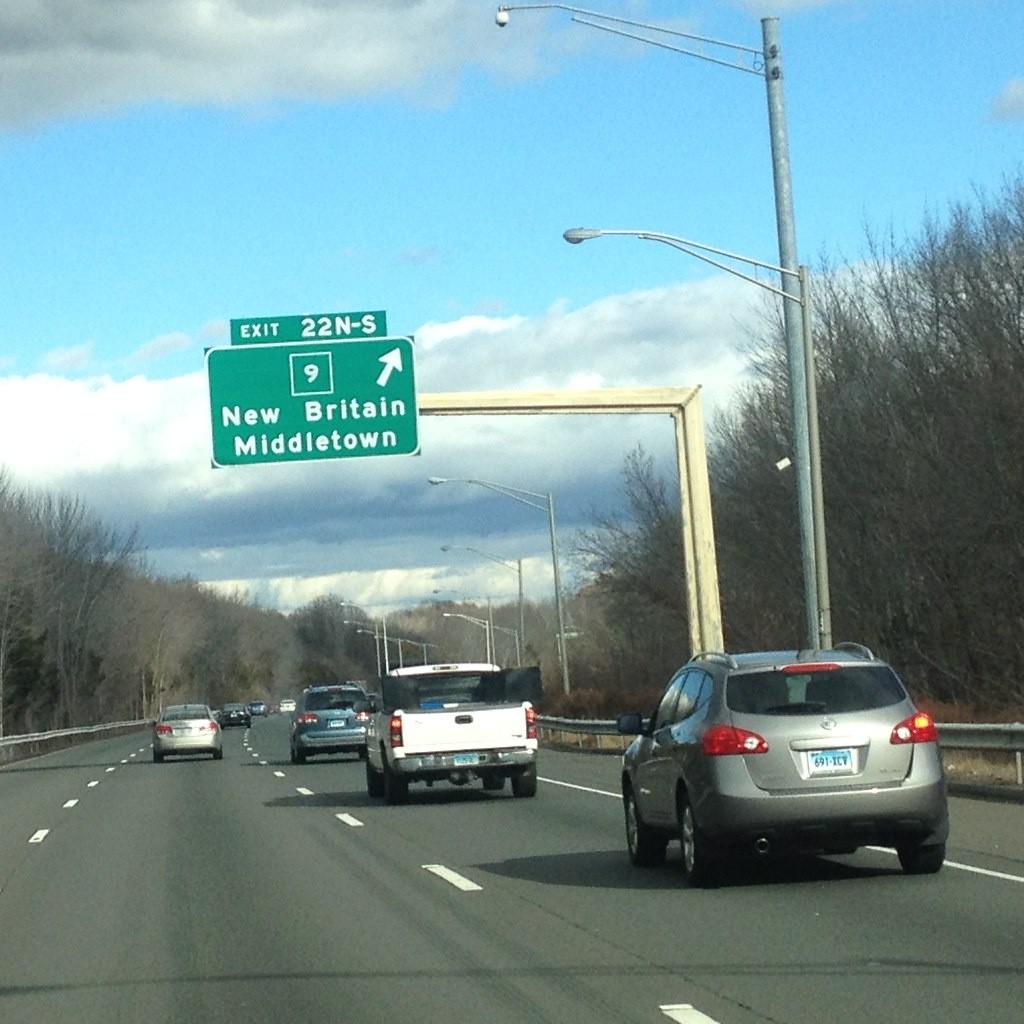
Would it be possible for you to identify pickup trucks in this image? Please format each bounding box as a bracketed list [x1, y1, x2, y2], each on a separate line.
[362, 664, 542, 797]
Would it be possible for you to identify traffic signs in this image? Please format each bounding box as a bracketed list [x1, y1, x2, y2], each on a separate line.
[207, 337, 422, 465]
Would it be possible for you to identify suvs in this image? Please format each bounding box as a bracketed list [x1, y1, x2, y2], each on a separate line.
[618, 639, 950, 876]
[292, 684, 377, 761]
[279, 699, 297, 715]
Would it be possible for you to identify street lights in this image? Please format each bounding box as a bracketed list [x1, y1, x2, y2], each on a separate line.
[358, 629, 381, 672]
[426, 475, 573, 697]
[433, 588, 497, 664]
[441, 546, 525, 666]
[374, 635, 403, 669]
[564, 228, 834, 658]
[341, 601, 390, 675]
[344, 619, 380, 673]
[441, 611, 490, 667]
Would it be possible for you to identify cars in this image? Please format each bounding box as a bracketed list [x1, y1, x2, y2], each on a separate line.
[267, 705, 280, 714]
[147, 705, 224, 760]
[249, 701, 267, 718]
[219, 702, 251, 728]
